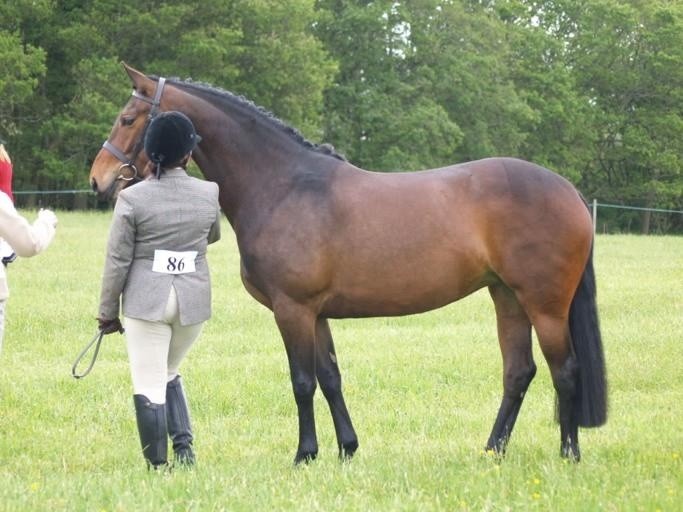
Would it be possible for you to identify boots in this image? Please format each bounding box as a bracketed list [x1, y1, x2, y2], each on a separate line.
[133, 375, 196, 470]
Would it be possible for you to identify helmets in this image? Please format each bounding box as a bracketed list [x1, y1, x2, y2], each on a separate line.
[145, 112, 195, 161]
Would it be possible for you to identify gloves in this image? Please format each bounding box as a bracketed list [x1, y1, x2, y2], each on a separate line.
[98, 320, 124, 334]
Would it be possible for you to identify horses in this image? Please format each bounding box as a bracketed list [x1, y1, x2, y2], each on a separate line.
[87, 60, 609, 466]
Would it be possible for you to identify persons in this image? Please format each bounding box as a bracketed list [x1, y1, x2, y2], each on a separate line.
[95, 110, 222, 473]
[0, 144, 58, 346]
